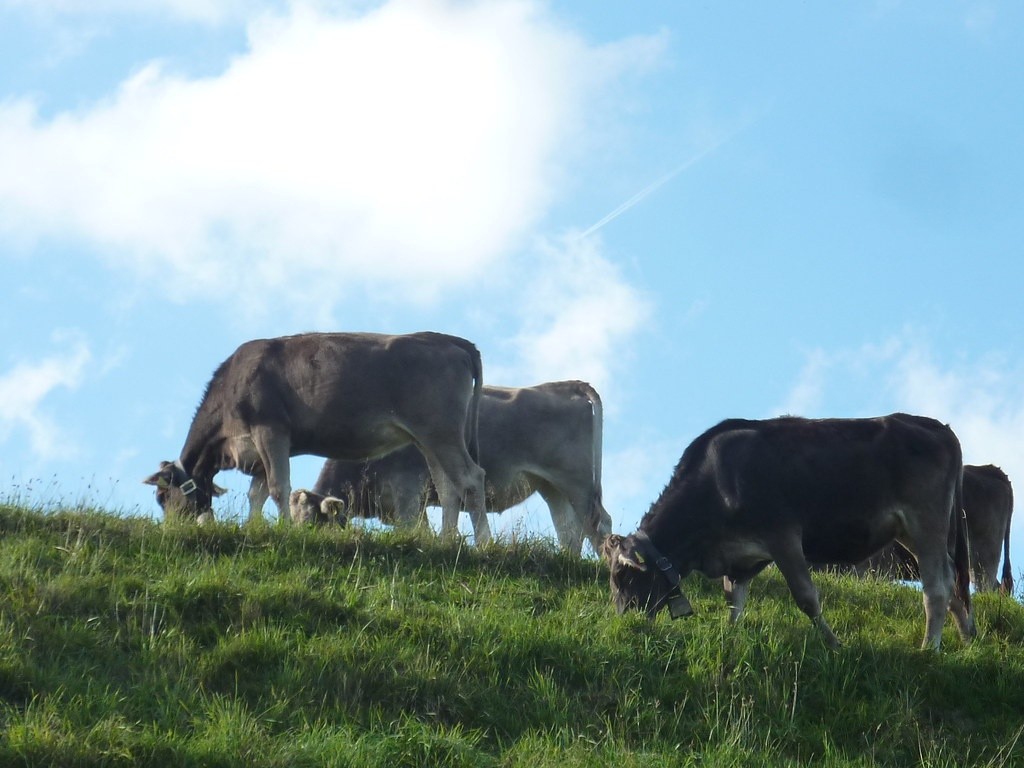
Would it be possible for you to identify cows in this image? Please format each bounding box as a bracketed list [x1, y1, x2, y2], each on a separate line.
[605, 411, 1014, 664]
[144, 330, 616, 563]
